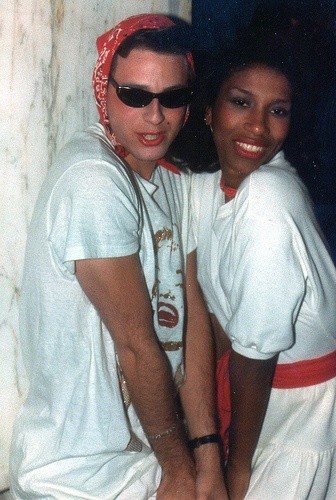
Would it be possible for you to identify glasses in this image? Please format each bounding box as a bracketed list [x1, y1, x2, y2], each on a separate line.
[108, 74, 197, 109]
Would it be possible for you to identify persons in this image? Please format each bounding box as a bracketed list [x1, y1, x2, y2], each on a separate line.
[182, 46, 336, 500]
[11, 12, 205, 500]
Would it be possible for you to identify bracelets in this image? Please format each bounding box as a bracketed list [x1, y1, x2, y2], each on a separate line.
[148, 421, 177, 441]
[189, 433, 218, 449]
